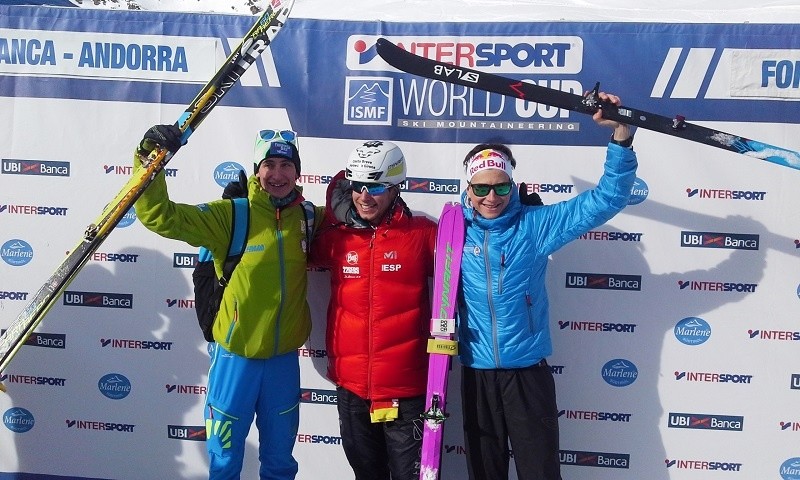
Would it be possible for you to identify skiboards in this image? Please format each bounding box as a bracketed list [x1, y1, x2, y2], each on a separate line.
[373, 37, 800, 171]
[416, 199, 466, 480]
[1, 0, 296, 372]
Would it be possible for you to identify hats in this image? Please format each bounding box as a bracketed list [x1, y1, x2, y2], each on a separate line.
[254, 131, 301, 175]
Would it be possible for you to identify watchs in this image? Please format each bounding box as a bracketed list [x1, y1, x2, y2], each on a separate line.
[610, 132, 634, 147]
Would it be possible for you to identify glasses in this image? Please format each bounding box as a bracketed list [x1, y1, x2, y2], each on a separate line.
[467, 178, 513, 197]
[256, 129, 300, 151]
[349, 181, 392, 195]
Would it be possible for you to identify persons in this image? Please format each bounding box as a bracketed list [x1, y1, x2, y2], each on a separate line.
[221, 140, 544, 480]
[458, 92, 639, 480]
[132, 125, 328, 480]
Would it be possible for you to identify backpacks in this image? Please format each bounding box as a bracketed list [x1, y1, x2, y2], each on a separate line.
[191, 198, 316, 342]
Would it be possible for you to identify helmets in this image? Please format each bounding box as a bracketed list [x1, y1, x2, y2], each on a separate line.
[344, 140, 407, 188]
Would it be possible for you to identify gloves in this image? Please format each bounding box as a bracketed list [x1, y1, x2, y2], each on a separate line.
[397, 196, 413, 217]
[221, 170, 249, 199]
[138, 125, 189, 157]
[519, 182, 544, 206]
